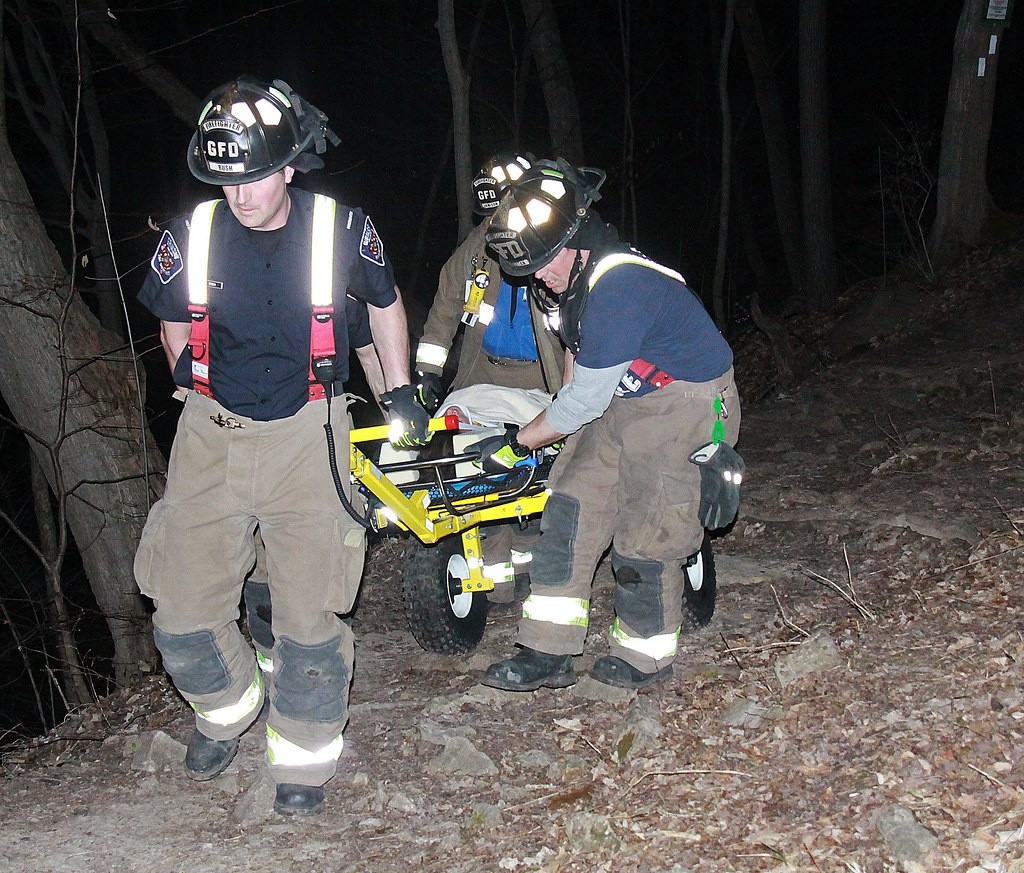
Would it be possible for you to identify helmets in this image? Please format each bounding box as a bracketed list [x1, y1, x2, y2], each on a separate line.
[472, 150, 539, 215]
[187, 78, 314, 187]
[486, 159, 605, 276]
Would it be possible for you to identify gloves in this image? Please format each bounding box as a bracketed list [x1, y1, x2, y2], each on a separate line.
[417, 372, 442, 409]
[462, 428, 531, 475]
[689, 442, 746, 531]
[378, 384, 434, 449]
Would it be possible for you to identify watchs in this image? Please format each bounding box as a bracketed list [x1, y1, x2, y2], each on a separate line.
[509, 434, 531, 457]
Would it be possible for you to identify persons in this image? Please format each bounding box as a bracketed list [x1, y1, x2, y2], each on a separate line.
[132, 82, 433, 810]
[415, 152, 565, 618]
[465, 159, 742, 692]
[247, 289, 392, 722]
[437, 384, 554, 427]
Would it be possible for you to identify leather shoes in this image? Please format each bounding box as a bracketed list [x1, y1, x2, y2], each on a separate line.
[274, 779, 325, 815]
[480, 643, 577, 689]
[590, 655, 675, 691]
[185, 732, 238, 782]
